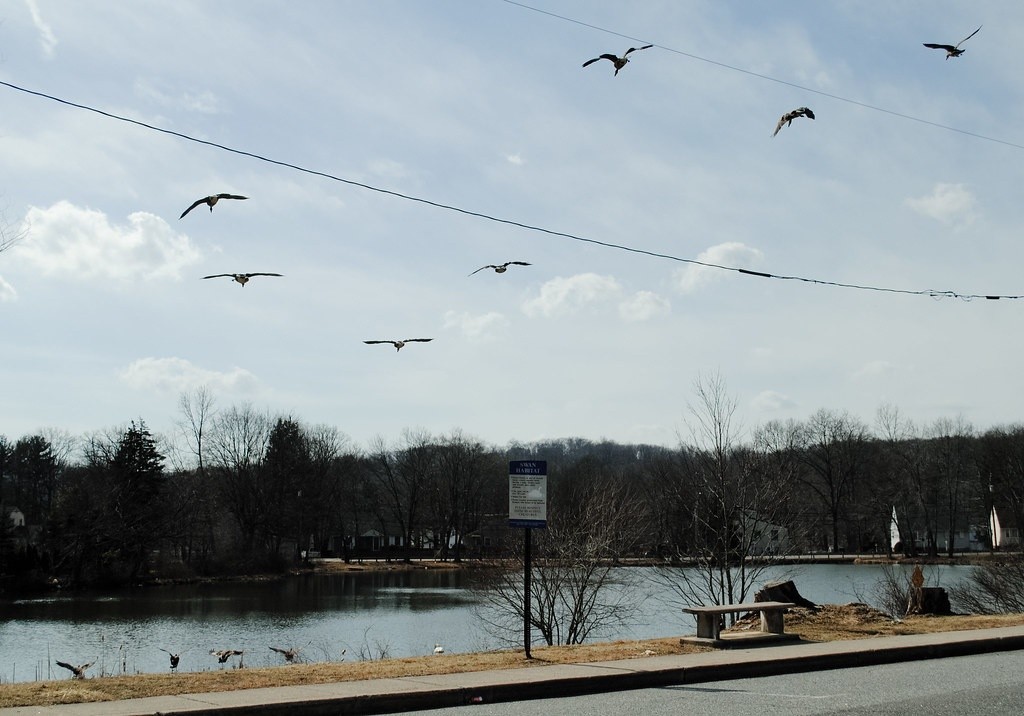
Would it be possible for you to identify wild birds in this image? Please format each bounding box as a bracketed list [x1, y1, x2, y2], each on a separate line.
[56, 655, 98, 679]
[269, 641, 312, 664]
[201, 272, 283, 288]
[208, 649, 243, 670]
[434, 644, 445, 654]
[582, 45, 653, 77]
[923, 24, 983, 61]
[161, 648, 190, 672]
[773, 107, 815, 136]
[364, 338, 433, 352]
[468, 261, 532, 276]
[178, 193, 249, 220]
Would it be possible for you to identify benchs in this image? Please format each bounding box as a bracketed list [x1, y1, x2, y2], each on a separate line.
[682, 601, 796, 640]
